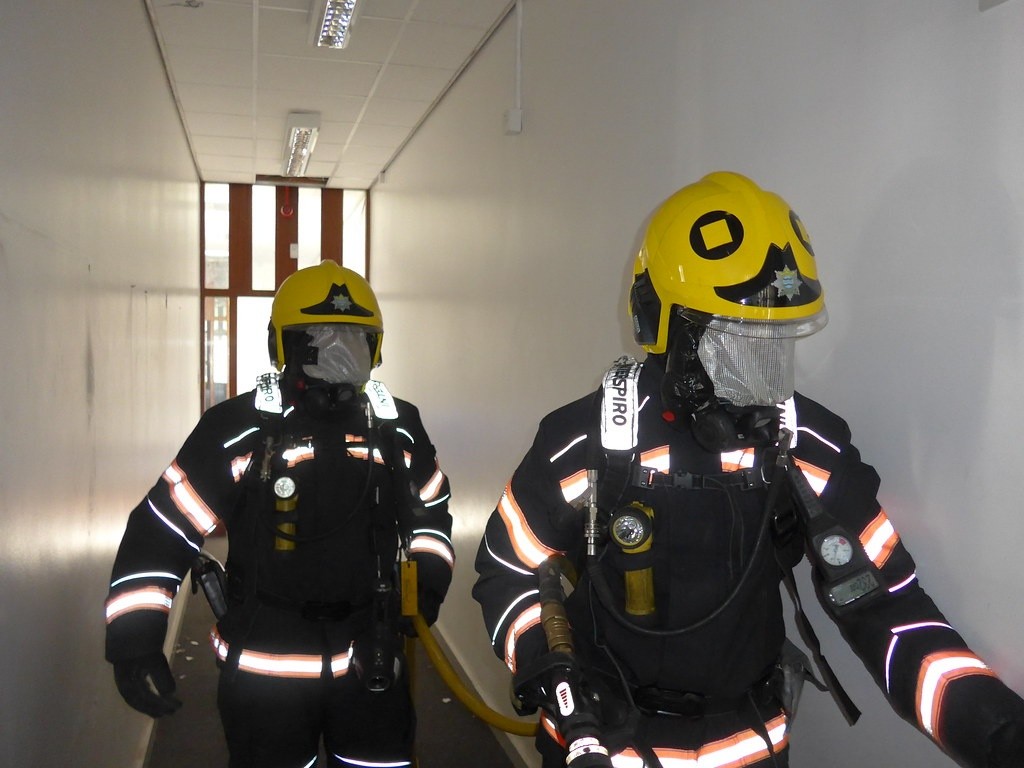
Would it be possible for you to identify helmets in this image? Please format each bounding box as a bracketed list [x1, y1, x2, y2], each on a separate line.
[623, 172, 830, 354]
[268, 260, 384, 370]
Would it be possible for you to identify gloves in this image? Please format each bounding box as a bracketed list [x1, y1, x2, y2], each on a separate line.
[939, 674, 1024, 768]
[390, 550, 452, 639]
[510, 624, 551, 716]
[103, 610, 184, 719]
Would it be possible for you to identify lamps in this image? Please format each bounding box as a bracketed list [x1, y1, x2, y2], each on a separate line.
[279, 113, 322, 179]
[305, 0, 363, 49]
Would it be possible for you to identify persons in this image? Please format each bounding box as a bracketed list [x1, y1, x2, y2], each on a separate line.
[472, 170, 1024, 768]
[104, 260, 456, 768]
